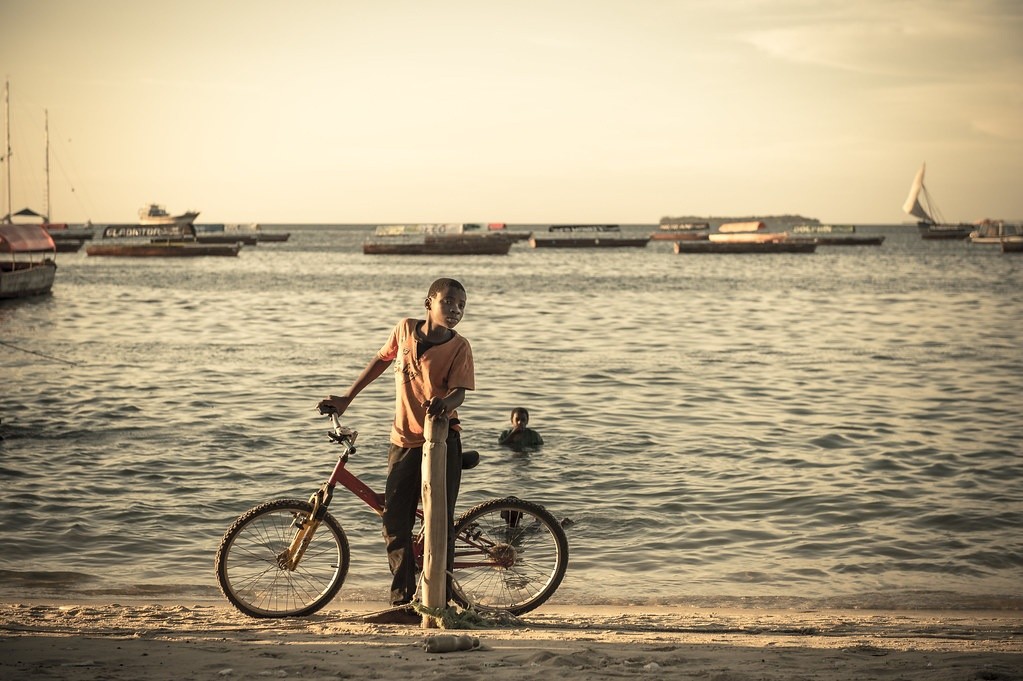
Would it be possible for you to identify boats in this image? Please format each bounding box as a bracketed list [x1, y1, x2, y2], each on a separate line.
[1001, 238, 1023, 253]
[138, 201, 225, 231]
[528, 223, 653, 248]
[650, 222, 710, 241]
[708, 221, 789, 242]
[373, 224, 531, 240]
[99, 211, 291, 246]
[362, 234, 517, 255]
[963, 218, 1023, 243]
[88, 241, 245, 258]
[788, 222, 887, 245]
[673, 242, 817, 253]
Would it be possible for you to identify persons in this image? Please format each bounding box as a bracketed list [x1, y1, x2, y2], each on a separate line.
[316, 278, 475, 607]
[498, 407, 544, 445]
[501, 496, 523, 527]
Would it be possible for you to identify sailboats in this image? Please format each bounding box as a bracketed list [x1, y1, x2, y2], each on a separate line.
[902, 159, 976, 239]
[0, 80, 57, 298]
[38, 106, 95, 254]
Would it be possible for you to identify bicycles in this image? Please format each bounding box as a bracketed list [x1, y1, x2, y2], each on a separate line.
[214, 405, 574, 620]
